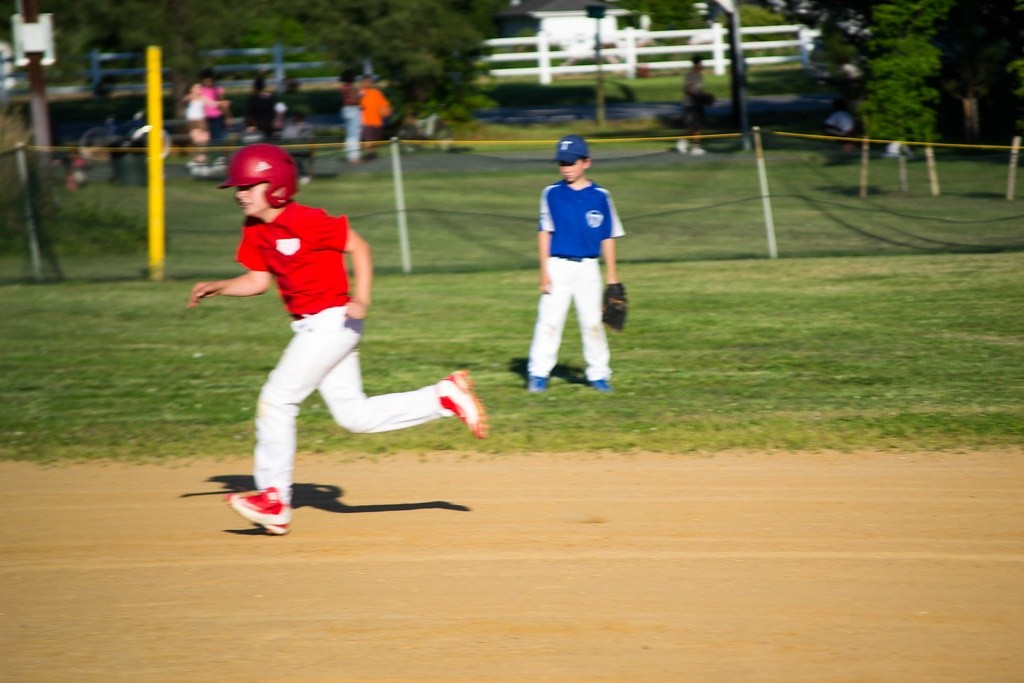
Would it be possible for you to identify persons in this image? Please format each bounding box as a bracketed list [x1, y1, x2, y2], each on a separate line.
[182, 65, 394, 179]
[185, 144, 492, 535]
[525, 134, 628, 393]
[672, 54, 717, 157]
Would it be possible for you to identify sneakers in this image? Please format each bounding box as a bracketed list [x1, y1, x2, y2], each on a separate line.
[226, 486, 292, 534]
[437, 370, 488, 439]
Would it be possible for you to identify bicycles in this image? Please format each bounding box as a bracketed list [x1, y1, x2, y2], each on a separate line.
[82, 104, 172, 164]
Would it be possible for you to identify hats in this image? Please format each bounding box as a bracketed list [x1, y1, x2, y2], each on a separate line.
[554, 135, 588, 163]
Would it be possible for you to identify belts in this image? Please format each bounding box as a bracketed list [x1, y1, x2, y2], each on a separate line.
[289, 311, 315, 321]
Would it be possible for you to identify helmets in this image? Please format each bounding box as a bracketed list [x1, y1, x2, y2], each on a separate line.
[217, 143, 298, 209]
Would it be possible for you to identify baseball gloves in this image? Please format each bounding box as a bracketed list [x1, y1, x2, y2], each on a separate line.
[601, 284, 628, 333]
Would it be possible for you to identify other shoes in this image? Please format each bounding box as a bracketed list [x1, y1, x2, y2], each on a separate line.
[689, 148, 706, 155]
[528, 376, 546, 392]
[677, 138, 688, 153]
[590, 380, 610, 391]
